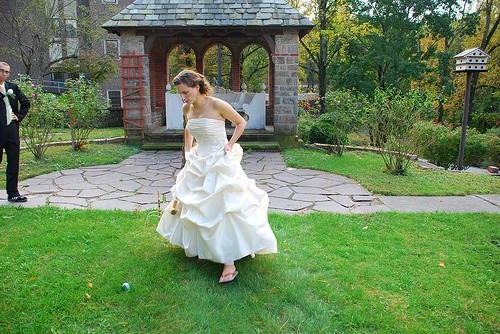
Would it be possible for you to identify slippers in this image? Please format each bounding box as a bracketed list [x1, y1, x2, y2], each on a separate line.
[218, 271, 238, 282]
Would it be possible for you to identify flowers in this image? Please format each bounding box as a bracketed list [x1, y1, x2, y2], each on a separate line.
[6, 89, 16, 100]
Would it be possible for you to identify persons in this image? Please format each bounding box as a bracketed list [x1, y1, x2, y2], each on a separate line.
[0, 61, 31, 201]
[156, 71, 278, 282]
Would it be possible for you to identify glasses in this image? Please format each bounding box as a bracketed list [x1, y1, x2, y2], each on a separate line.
[0, 68, 10, 74]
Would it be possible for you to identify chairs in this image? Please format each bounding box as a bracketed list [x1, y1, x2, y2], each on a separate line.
[165, 83, 266, 130]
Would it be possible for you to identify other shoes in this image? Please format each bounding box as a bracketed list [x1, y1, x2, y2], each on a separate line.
[8, 194, 27, 202]
